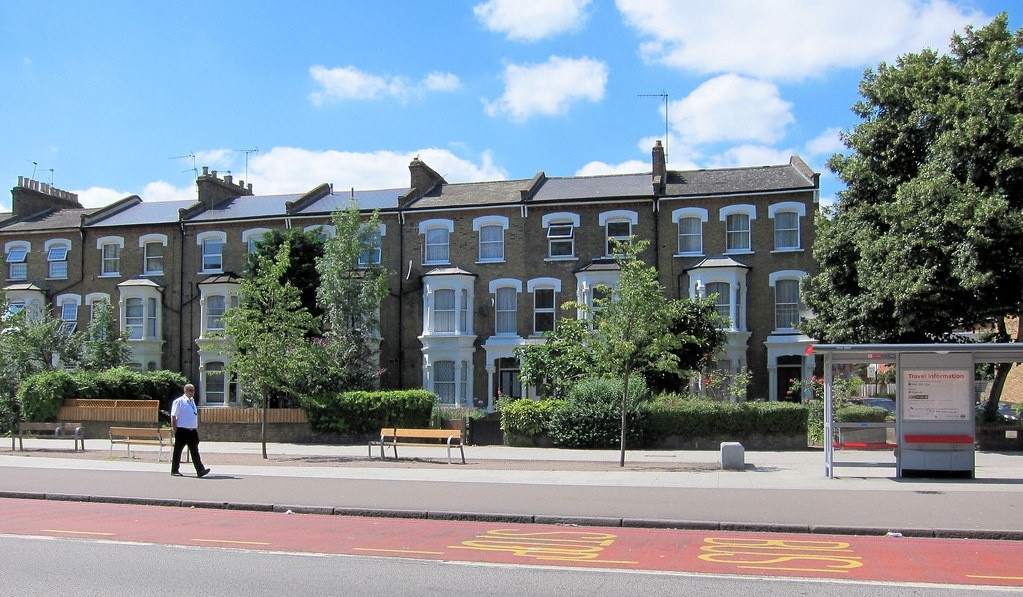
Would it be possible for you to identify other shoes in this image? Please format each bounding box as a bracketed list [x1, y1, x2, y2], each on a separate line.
[198, 468, 210, 477]
[171, 472, 183, 476]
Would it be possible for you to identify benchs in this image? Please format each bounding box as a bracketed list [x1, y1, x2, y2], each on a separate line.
[109, 427, 189, 464]
[832, 441, 897, 451]
[368, 428, 466, 465]
[12, 421, 85, 452]
[903, 435, 974, 443]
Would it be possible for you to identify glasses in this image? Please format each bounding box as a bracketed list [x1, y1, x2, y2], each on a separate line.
[186, 389, 195, 392]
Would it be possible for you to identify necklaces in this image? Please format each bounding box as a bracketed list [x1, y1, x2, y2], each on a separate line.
[188, 401, 197, 415]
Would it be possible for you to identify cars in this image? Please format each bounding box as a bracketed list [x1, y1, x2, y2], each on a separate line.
[847, 397, 896, 410]
[975, 400, 1020, 421]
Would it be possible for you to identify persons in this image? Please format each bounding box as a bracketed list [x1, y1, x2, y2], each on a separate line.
[170, 383, 210, 478]
